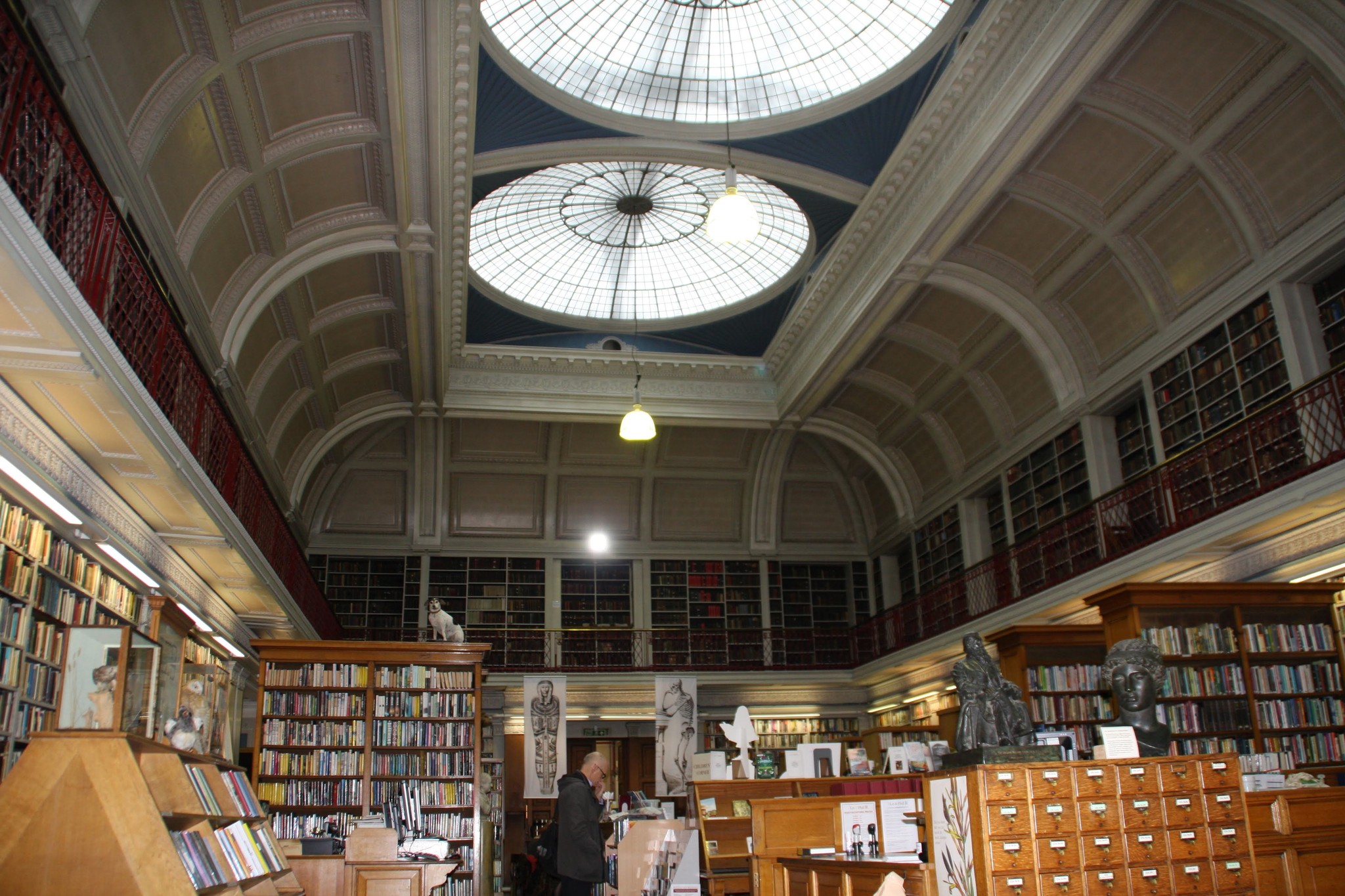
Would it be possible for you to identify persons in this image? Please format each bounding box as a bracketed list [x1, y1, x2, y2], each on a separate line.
[558, 751, 609, 896]
[1100, 639, 1171, 758]
[951, 631, 1038, 753]
[480, 773, 493, 822]
[528, 800, 562, 896]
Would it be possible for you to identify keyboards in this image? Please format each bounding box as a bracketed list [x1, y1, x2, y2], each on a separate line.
[414, 852, 439, 861]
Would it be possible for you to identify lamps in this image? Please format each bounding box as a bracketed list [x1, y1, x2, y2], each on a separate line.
[618, 213, 657, 442]
[708, 124, 761, 245]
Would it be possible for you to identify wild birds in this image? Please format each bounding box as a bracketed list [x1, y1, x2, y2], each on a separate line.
[164, 706, 204, 754]
[179, 677, 212, 755]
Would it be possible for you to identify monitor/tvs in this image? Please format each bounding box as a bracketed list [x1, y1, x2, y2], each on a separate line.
[382, 780, 421, 848]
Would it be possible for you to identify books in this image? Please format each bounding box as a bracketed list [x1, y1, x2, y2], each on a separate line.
[891, 266, 1345, 638]
[733, 800, 751, 817]
[700, 797, 717, 818]
[746, 836, 752, 853]
[0, 492, 229, 784]
[169, 820, 286, 890]
[309, 553, 875, 668]
[433, 877, 472, 896]
[847, 748, 872, 776]
[706, 841, 718, 856]
[1141, 609, 1345, 792]
[874, 691, 960, 774]
[1027, 664, 1116, 761]
[259, 663, 475, 872]
[184, 764, 260, 816]
[590, 790, 650, 896]
[704, 718, 861, 779]
[797, 847, 836, 856]
[481, 726, 501, 892]
[641, 841, 687, 896]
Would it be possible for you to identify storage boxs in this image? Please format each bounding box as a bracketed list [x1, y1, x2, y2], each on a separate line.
[53, 625, 162, 740]
[693, 752, 726, 780]
[179, 663, 231, 759]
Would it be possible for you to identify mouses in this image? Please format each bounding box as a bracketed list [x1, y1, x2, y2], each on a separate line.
[444, 852, 461, 860]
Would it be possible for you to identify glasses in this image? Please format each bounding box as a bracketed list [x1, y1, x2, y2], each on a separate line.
[594, 764, 606, 779]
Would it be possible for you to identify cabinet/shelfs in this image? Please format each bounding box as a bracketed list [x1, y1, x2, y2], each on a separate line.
[249, 639, 504, 896]
[861, 725, 939, 773]
[0, 471, 302, 896]
[937, 707, 960, 752]
[984, 624, 1113, 759]
[874, 688, 960, 727]
[685, 774, 918, 896]
[309, 557, 869, 668]
[921, 752, 1261, 896]
[870, 264, 1345, 659]
[1082, 582, 1345, 786]
[704, 716, 860, 766]
[604, 820, 685, 896]
[828, 737, 863, 776]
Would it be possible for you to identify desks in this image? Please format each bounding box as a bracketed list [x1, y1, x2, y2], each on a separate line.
[1246, 785, 1345, 896]
[776, 854, 931, 896]
[286, 855, 464, 896]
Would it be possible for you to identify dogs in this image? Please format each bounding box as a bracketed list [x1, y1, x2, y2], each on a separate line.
[423, 597, 466, 643]
